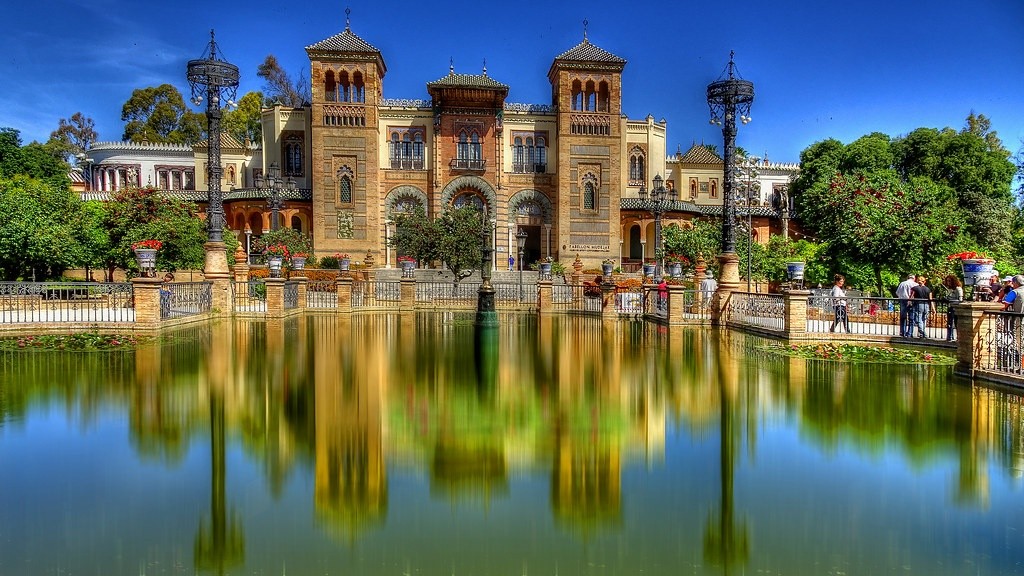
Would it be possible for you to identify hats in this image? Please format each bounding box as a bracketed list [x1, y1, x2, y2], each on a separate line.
[1002, 276, 1012, 282]
[990, 270, 999, 277]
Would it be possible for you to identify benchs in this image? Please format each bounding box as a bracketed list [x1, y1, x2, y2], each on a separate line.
[0, 295, 43, 311]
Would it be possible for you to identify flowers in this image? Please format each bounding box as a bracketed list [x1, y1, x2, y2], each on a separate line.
[263, 244, 289, 262]
[292, 252, 309, 258]
[603, 259, 616, 265]
[335, 253, 353, 261]
[946, 249, 998, 263]
[398, 255, 417, 264]
[130, 240, 163, 251]
[642, 260, 656, 266]
[665, 254, 691, 266]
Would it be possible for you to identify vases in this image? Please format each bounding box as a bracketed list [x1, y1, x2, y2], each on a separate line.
[401, 261, 415, 271]
[541, 263, 551, 275]
[668, 262, 683, 277]
[602, 264, 614, 276]
[293, 259, 306, 269]
[339, 260, 350, 271]
[644, 265, 655, 277]
[266, 255, 283, 275]
[134, 249, 157, 268]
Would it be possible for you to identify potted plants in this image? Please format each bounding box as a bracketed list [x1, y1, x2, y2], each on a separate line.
[963, 259, 994, 286]
[784, 257, 806, 280]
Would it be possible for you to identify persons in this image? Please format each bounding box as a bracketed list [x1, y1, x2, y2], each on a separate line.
[945, 274, 963, 339]
[983, 270, 1024, 374]
[160, 273, 174, 317]
[701, 270, 717, 308]
[658, 279, 667, 311]
[508, 255, 515, 271]
[907, 276, 936, 336]
[896, 274, 919, 336]
[830, 274, 850, 333]
